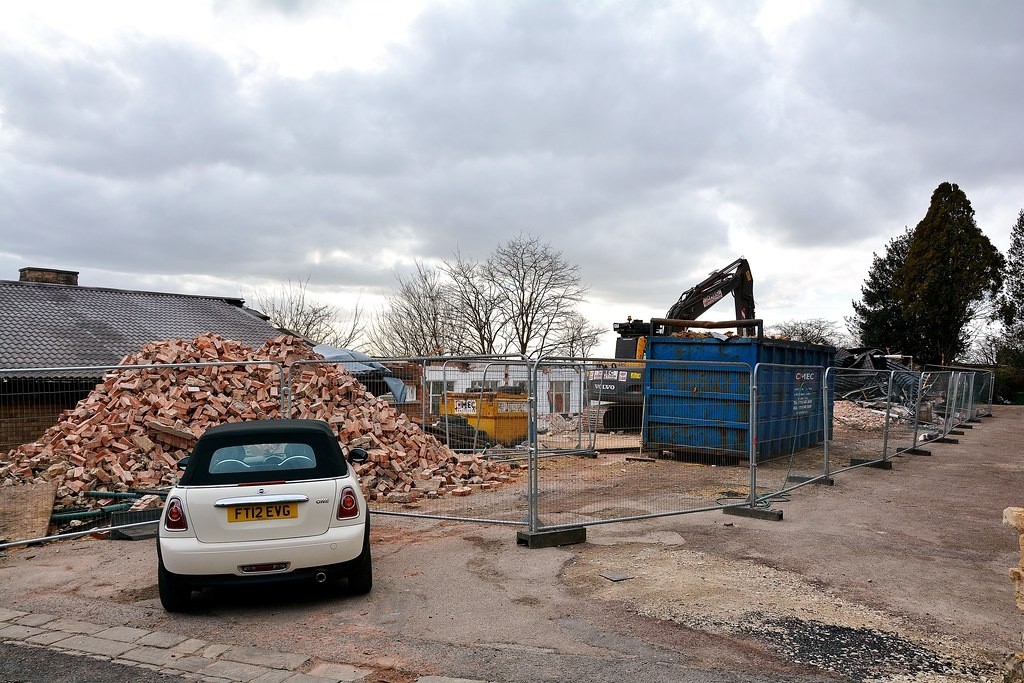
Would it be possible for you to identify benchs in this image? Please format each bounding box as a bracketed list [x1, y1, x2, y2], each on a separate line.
[212, 456, 316, 471]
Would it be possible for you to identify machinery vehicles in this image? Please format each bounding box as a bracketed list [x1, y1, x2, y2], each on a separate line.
[581, 254, 756, 434]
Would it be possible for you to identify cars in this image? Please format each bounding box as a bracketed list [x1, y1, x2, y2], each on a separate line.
[156, 418, 371, 612]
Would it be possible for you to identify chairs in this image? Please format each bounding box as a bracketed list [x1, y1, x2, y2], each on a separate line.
[284, 444, 313, 458]
[217, 445, 246, 461]
